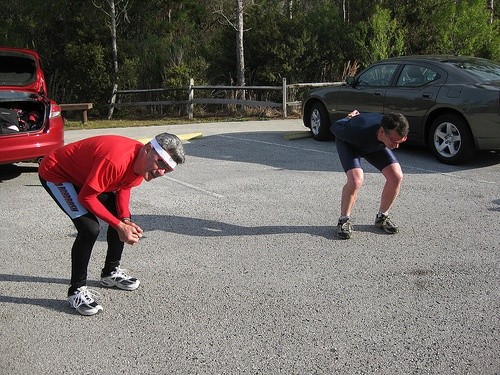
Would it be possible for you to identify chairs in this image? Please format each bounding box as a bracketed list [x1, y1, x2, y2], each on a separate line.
[402, 67, 424, 86]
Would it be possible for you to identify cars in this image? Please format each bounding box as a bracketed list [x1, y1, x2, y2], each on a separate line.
[301, 54, 500, 165]
[0, 47, 66, 167]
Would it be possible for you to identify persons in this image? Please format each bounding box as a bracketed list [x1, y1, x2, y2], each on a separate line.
[36, 132, 187, 316]
[328, 109, 410, 240]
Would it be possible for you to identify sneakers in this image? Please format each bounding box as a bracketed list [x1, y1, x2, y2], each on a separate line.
[337, 217, 353, 239]
[375, 214, 399, 234]
[67, 285, 103, 315]
[100, 265, 141, 292]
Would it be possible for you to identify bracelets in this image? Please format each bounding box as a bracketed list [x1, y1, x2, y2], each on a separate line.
[121, 218, 131, 222]
